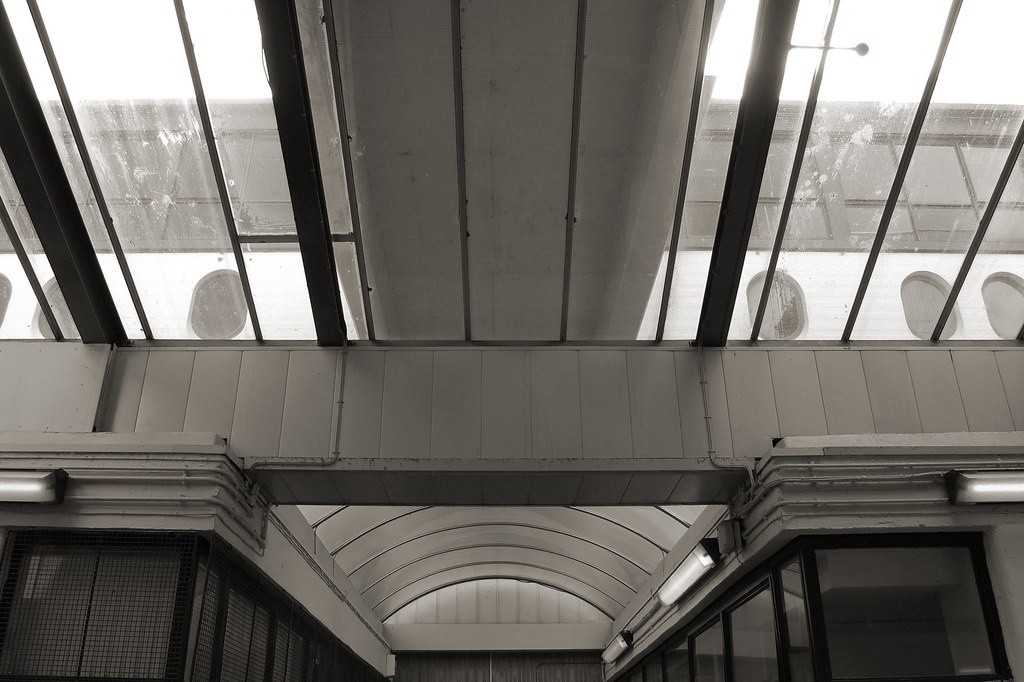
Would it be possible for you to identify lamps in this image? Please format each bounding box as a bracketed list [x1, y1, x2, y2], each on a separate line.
[657, 537, 722, 606]
[602, 630, 634, 664]
[942, 468, 1024, 505]
[0, 467, 69, 504]
